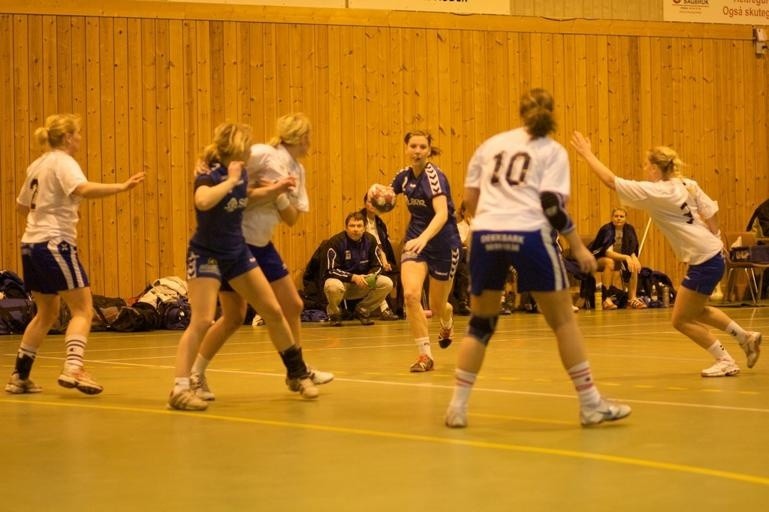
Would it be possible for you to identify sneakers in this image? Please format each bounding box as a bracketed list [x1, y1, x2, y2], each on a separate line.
[457, 298, 579, 314]
[438, 318, 454, 348]
[329, 307, 397, 326]
[604, 297, 647, 310]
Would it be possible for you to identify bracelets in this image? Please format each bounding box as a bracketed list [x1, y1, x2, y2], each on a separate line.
[272, 194, 291, 211]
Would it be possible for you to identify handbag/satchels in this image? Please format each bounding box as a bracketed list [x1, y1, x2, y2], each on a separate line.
[106, 307, 161, 331]
[0, 298, 36, 335]
[91, 295, 126, 332]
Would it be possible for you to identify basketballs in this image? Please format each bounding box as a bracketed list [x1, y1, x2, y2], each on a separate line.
[372, 185, 397, 213]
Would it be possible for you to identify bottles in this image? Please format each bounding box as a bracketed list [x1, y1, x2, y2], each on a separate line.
[651, 284, 658, 302]
[662, 287, 670, 307]
[594, 287, 603, 310]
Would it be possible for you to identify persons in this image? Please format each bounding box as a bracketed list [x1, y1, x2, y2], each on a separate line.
[190, 111, 336, 402]
[569, 127, 763, 379]
[364, 130, 464, 374]
[168, 118, 320, 411]
[322, 192, 399, 326]
[453, 201, 535, 314]
[442, 86, 633, 430]
[741, 198, 769, 301]
[2, 112, 149, 397]
[559, 207, 646, 309]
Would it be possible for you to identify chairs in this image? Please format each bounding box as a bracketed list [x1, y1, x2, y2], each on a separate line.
[722, 230, 768, 307]
